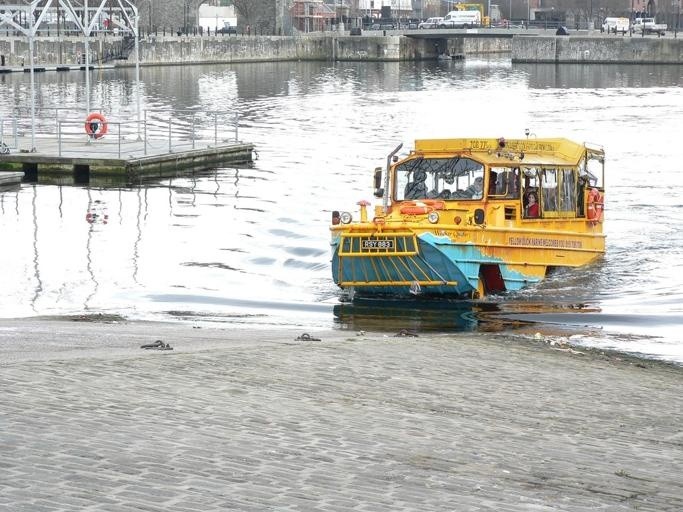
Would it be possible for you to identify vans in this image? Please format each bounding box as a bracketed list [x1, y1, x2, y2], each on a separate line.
[601, 17, 629, 34]
[436, 9, 482, 29]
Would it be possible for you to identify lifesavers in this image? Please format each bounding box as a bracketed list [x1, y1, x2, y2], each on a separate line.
[401, 198, 444, 215]
[586, 188, 602, 225]
[84, 112, 107, 139]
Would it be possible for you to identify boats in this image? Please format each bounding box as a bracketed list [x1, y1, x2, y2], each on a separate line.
[328, 128, 605, 300]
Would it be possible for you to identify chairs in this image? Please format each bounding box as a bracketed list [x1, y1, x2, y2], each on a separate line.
[521, 187, 567, 216]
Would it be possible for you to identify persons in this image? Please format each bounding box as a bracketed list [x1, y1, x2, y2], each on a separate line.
[404, 164, 588, 221]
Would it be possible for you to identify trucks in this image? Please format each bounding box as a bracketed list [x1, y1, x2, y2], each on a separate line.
[631, 17, 667, 34]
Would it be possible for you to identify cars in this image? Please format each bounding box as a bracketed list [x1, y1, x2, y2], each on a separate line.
[417, 15, 445, 30]
